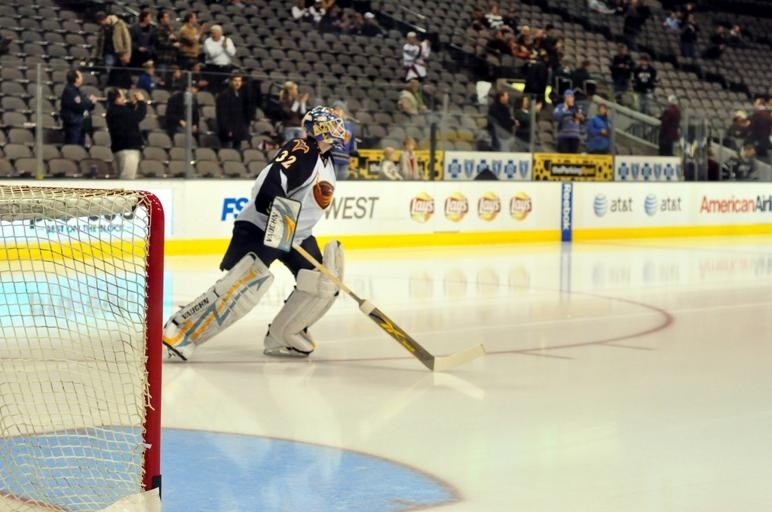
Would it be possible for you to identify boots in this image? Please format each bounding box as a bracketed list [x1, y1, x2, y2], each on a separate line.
[265, 330, 315, 354]
[159, 328, 194, 360]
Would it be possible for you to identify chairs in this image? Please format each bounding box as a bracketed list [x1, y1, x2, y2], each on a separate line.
[0, 1, 771, 185]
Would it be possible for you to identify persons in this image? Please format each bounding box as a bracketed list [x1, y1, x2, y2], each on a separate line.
[338, 1, 385, 36]
[106, 85, 149, 180]
[59, 69, 97, 146]
[163, 106, 344, 361]
[724, 96, 772, 166]
[376, 144, 404, 181]
[399, 137, 421, 181]
[609, 43, 657, 115]
[93, 1, 361, 150]
[722, 136, 760, 181]
[329, 103, 360, 180]
[461, 5, 612, 155]
[395, 32, 444, 116]
[584, 0, 772, 60]
[657, 94, 680, 156]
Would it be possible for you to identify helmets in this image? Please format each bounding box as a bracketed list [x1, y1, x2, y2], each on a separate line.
[301, 105, 346, 144]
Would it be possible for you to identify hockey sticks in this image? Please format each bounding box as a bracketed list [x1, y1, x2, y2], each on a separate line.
[292, 239, 489, 372]
